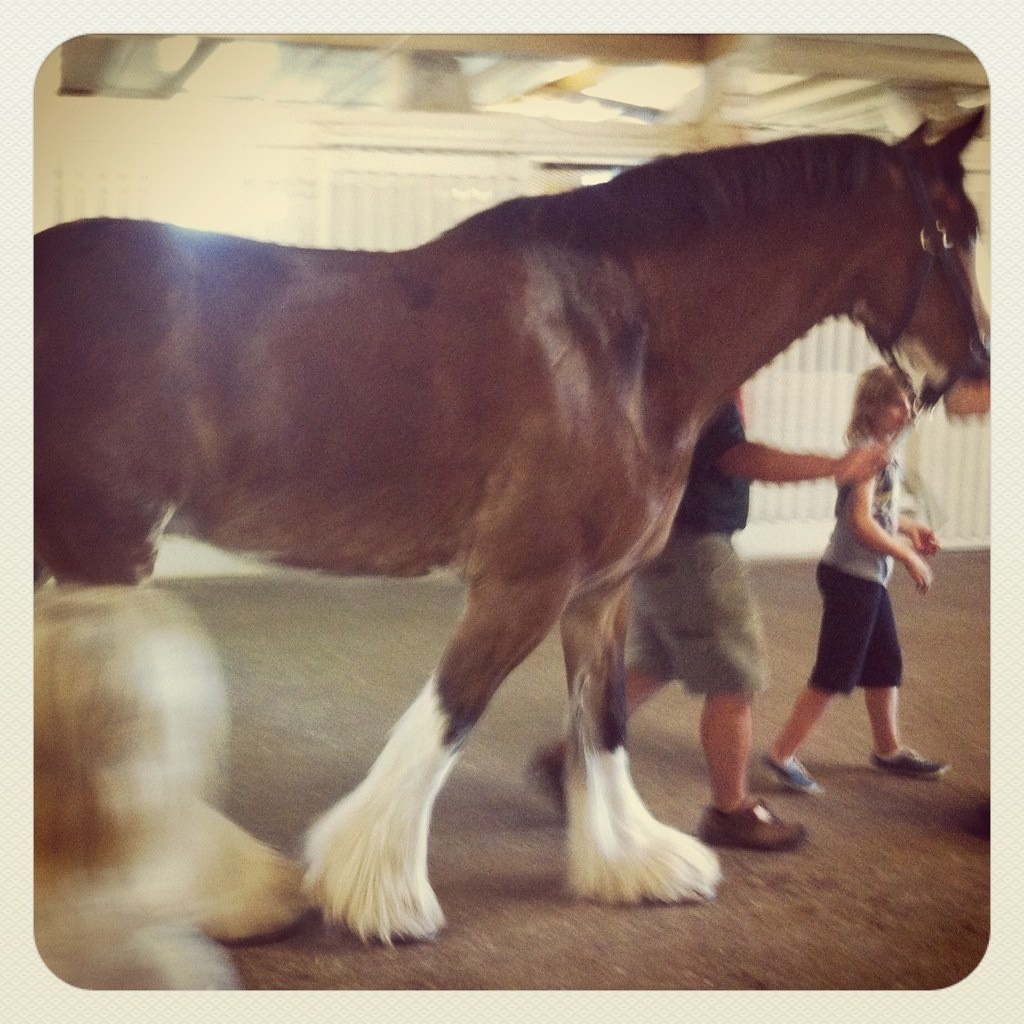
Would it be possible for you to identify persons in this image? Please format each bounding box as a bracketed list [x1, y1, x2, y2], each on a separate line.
[527, 388, 887, 850]
[762, 364, 949, 795]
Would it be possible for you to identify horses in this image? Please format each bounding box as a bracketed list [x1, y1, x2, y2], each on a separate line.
[34, 102, 991, 944]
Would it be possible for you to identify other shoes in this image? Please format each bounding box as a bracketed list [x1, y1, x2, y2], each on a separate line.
[534, 745, 564, 809]
[700, 802, 804, 851]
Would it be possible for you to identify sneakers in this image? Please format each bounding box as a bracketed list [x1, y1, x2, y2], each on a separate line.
[762, 755, 824, 794]
[871, 742, 949, 778]
